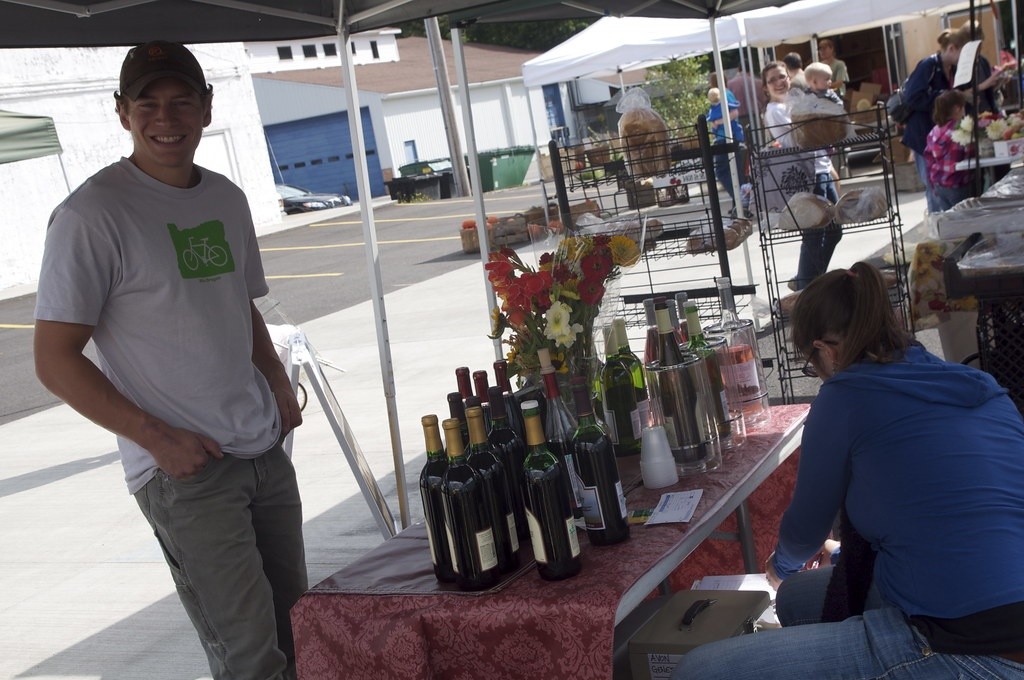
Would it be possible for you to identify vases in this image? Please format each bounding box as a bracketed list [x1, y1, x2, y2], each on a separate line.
[978, 137, 994, 158]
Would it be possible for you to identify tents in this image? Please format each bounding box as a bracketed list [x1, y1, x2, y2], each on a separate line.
[522, 1, 779, 230]
[0, 1, 792, 530]
[743, 1, 1017, 151]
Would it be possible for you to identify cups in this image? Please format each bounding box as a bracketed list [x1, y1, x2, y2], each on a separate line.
[640, 425, 678, 490]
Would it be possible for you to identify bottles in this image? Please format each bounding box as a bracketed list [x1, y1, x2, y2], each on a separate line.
[681, 300, 733, 440]
[602, 325, 642, 456]
[666, 299, 683, 345]
[713, 276, 761, 415]
[613, 317, 652, 431]
[641, 299, 663, 417]
[675, 292, 689, 343]
[654, 296, 706, 463]
[417, 343, 631, 594]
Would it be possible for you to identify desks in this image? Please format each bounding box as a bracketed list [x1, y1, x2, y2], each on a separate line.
[289, 402, 811, 680]
[955, 154, 1023, 194]
[946, 228, 1024, 412]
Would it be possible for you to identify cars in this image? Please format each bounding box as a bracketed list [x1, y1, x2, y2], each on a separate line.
[276, 183, 354, 216]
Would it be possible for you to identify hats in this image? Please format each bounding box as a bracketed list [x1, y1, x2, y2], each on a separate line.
[120, 39, 206, 102]
[817, 40, 832, 49]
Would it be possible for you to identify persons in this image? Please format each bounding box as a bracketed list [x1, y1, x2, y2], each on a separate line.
[888, 21, 1016, 217]
[766, 37, 849, 289]
[34, 43, 312, 680]
[708, 61, 769, 218]
[670, 262, 1024, 680]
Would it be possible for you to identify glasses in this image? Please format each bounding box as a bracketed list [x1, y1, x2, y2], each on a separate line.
[802, 338, 839, 378]
[766, 75, 789, 85]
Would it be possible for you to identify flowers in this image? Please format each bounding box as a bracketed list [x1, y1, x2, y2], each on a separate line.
[951, 111, 1024, 145]
[484, 223, 642, 403]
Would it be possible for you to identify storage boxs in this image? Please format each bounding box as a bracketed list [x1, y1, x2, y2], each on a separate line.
[844, 83, 926, 193]
[994, 138, 1024, 157]
[628, 589, 770, 680]
[461, 200, 603, 253]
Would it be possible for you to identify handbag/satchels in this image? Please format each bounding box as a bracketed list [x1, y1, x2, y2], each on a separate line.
[887, 53, 936, 125]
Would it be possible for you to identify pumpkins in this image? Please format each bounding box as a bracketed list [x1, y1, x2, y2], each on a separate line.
[461, 215, 516, 230]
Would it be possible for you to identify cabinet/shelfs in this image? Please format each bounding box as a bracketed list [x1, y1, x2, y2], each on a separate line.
[548, 115, 758, 344]
[742, 101, 917, 404]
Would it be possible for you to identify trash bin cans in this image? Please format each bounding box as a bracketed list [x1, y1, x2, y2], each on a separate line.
[399, 145, 536, 192]
[384, 171, 458, 204]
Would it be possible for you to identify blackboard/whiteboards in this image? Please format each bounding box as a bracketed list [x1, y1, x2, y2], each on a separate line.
[281, 330, 398, 542]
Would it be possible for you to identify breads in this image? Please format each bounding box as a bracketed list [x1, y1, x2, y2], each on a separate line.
[619, 107, 670, 174]
[790, 111, 849, 150]
[686, 219, 750, 254]
[834, 189, 885, 223]
[777, 192, 833, 230]
